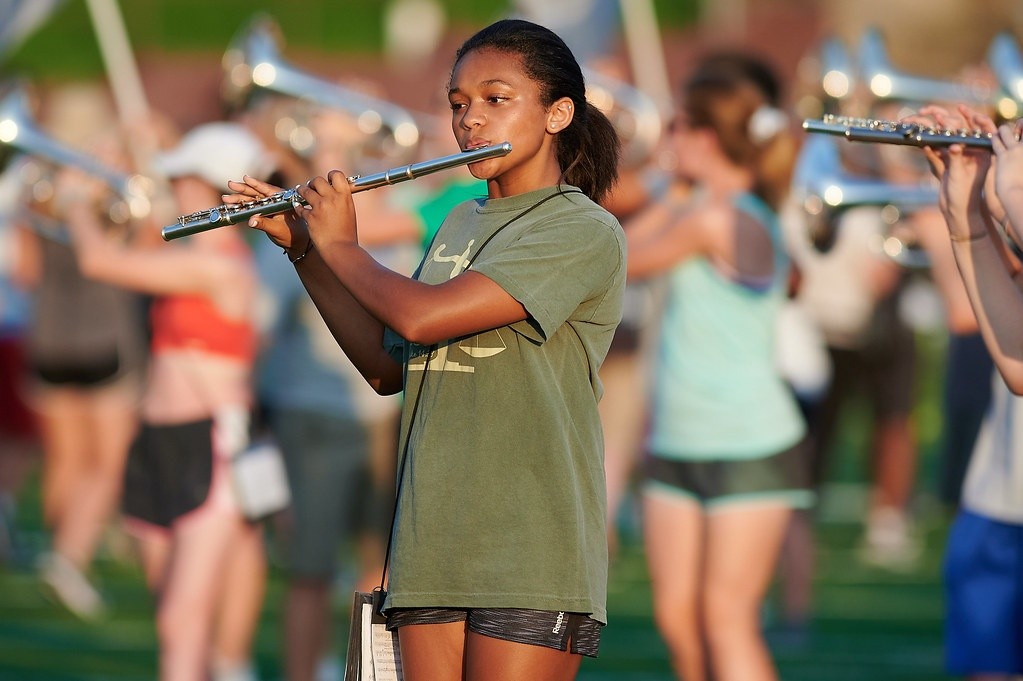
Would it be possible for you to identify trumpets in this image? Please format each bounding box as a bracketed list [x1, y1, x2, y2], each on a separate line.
[3, 15, 416, 234]
[793, 28, 1022, 212]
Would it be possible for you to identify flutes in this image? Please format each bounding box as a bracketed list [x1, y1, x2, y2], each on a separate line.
[801, 115, 995, 151]
[162, 144, 512, 243]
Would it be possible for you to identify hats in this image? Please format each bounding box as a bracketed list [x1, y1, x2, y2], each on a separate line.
[151, 120, 276, 194]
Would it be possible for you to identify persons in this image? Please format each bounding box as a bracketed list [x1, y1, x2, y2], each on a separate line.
[0, 49, 1023, 681]
[221, 18, 628, 681]
[900, 103, 1023, 397]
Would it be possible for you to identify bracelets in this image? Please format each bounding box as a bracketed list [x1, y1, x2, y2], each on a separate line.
[283, 239, 313, 265]
[950, 230, 988, 242]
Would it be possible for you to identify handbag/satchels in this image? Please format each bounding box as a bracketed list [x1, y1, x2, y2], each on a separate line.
[229, 442, 292, 522]
